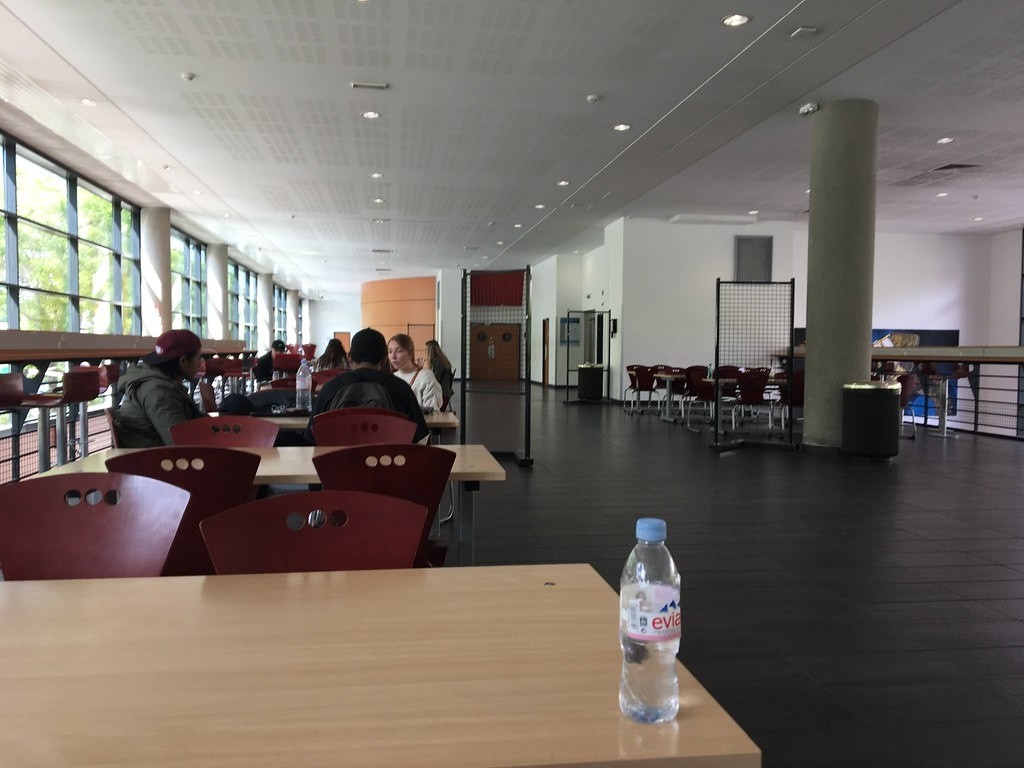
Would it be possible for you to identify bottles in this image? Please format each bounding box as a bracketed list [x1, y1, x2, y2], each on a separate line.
[298, 345, 304, 355]
[707, 362, 713, 380]
[620, 518, 680, 724]
[297, 359, 312, 410]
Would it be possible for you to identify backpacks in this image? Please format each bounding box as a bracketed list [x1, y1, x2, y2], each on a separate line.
[326, 371, 395, 411]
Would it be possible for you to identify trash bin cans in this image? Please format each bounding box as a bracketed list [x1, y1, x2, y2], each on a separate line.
[578, 364, 604, 403]
[840, 381, 902, 459]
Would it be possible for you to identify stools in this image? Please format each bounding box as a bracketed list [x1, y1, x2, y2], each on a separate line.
[871, 363, 906, 381]
[0, 354, 258, 484]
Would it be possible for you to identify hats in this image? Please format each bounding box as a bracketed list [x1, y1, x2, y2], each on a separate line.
[272, 340, 286, 352]
[142, 329, 201, 366]
[351, 327, 386, 358]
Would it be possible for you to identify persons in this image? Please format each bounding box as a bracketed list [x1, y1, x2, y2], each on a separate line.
[303, 328, 431, 492]
[112, 329, 273, 500]
[251, 339, 350, 401]
[381, 334, 452, 445]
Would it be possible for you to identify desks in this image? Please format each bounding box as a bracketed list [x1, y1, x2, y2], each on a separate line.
[702, 377, 786, 434]
[923, 369, 969, 441]
[209, 412, 460, 442]
[21, 444, 505, 540]
[656, 372, 686, 425]
[260, 384, 325, 393]
[0, 563, 761, 768]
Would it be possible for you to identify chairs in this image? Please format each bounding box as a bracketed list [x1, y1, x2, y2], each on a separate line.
[623, 364, 918, 448]
[0, 368, 457, 581]
